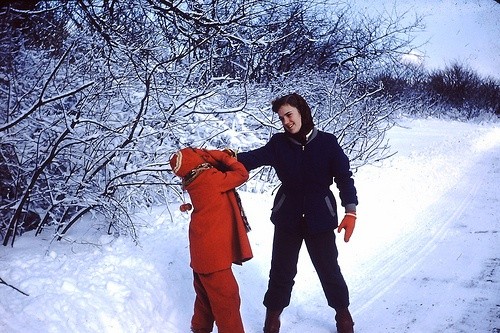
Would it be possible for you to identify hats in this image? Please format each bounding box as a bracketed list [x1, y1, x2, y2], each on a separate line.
[167, 149, 205, 180]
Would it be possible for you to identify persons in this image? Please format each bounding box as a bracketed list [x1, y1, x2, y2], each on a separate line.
[169, 147, 254, 333]
[223, 94, 358, 333]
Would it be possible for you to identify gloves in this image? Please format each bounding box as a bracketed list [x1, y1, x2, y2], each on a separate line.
[193, 145, 221, 167]
[336, 209, 358, 242]
[210, 148, 239, 168]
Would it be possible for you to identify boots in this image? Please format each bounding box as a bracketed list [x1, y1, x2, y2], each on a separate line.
[332, 304, 355, 333]
[262, 305, 284, 333]
